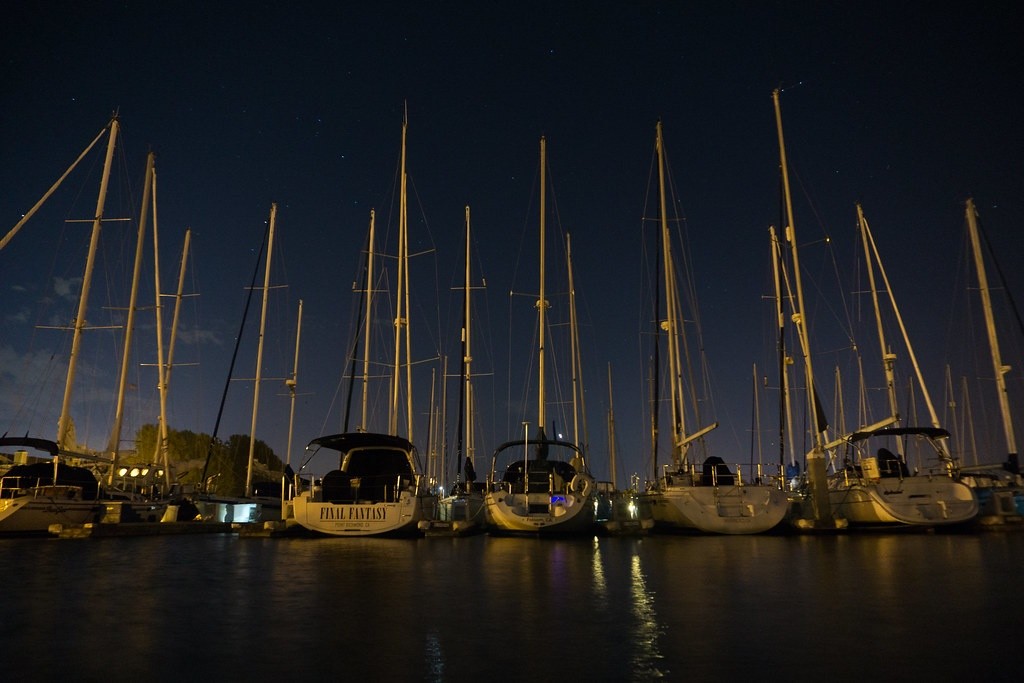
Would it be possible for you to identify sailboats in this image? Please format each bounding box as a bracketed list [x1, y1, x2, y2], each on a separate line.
[0, 84, 1023, 539]
